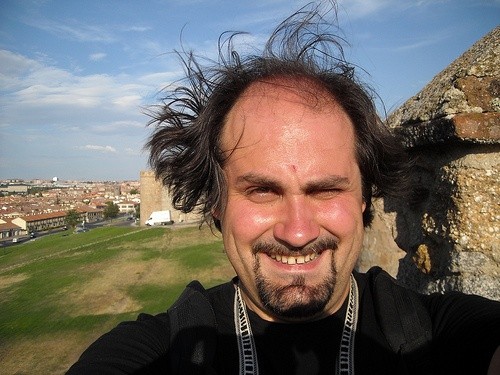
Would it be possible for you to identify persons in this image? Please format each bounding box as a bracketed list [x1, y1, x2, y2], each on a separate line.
[64, 0, 500, 375]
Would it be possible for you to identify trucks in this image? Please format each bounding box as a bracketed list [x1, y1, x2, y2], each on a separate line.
[144, 210, 171, 226]
